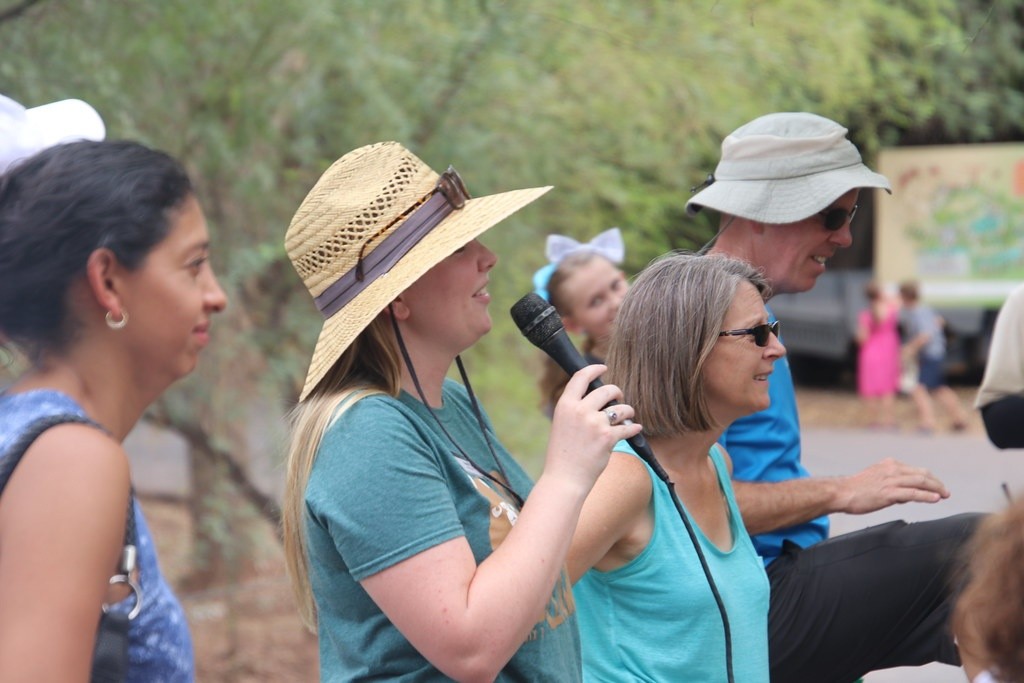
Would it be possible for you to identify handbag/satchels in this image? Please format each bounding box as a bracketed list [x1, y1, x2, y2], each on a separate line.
[899, 353, 918, 395]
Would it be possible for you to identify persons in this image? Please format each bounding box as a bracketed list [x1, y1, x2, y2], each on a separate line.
[565, 251, 786, 683]
[0, 136, 227, 683]
[284, 140, 644, 683]
[851, 274, 1024, 683]
[529, 226, 631, 419]
[687, 111, 999, 683]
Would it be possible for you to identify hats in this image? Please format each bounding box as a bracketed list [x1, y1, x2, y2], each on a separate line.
[284, 142, 555, 402]
[0, 93, 106, 177]
[683, 112, 893, 224]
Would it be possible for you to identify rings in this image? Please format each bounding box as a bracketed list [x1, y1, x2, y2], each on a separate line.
[604, 408, 618, 426]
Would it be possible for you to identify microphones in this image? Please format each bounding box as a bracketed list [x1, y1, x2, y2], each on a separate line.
[510, 291, 671, 481]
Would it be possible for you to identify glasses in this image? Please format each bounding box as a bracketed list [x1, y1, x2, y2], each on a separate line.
[719, 320, 779, 347]
[356, 164, 472, 282]
[820, 205, 858, 231]
